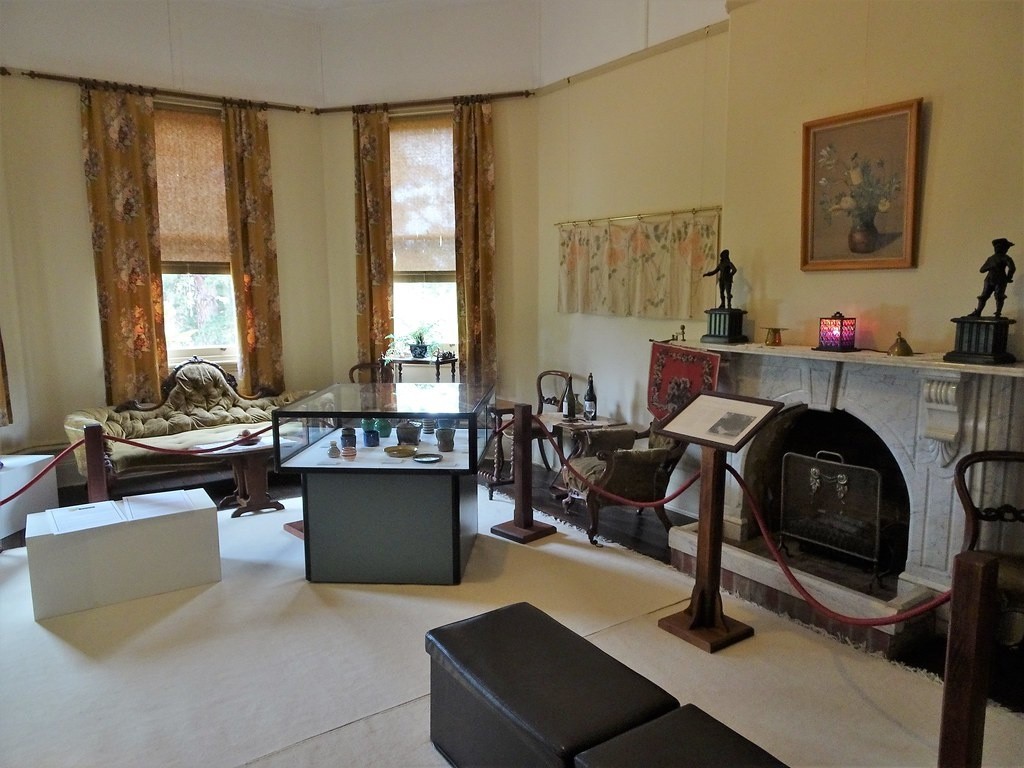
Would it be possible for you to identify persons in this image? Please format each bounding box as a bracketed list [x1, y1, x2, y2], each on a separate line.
[703, 249, 738, 310]
[968, 238, 1016, 317]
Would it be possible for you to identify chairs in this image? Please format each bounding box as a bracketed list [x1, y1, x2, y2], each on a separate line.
[562, 417, 690, 547]
[952, 451, 1024, 657]
[349, 362, 395, 412]
[487, 371, 577, 501]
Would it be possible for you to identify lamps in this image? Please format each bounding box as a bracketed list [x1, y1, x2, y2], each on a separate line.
[811, 311, 863, 354]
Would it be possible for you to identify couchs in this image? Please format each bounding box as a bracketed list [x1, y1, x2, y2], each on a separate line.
[64, 359, 335, 504]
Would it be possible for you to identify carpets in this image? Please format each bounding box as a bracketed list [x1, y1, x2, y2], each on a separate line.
[0, 479, 1024, 768]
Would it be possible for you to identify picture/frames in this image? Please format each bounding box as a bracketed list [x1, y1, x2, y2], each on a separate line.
[800, 97, 923, 271]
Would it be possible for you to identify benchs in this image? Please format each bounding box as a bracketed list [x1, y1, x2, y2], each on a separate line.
[574, 703, 791, 768]
[424, 601, 679, 768]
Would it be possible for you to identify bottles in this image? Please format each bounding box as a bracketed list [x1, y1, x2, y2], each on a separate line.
[328, 441, 340, 458]
[374, 418, 391, 438]
[341, 428, 356, 449]
[584, 372, 597, 421]
[563, 377, 576, 422]
[361, 417, 376, 431]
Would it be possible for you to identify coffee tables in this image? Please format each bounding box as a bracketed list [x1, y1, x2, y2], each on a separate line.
[189, 435, 296, 518]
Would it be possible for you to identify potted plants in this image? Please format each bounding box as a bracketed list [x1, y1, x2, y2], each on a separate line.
[382, 320, 440, 368]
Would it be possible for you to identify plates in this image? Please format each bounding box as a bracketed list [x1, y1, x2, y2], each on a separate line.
[383, 445, 418, 458]
[413, 453, 443, 462]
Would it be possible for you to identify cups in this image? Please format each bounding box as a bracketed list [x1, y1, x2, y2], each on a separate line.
[363, 430, 379, 447]
[342, 447, 356, 461]
[421, 418, 434, 434]
[436, 428, 456, 452]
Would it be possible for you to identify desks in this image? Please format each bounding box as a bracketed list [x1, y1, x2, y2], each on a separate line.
[27, 487, 222, 623]
[534, 413, 625, 498]
[478, 399, 520, 483]
[378, 357, 459, 384]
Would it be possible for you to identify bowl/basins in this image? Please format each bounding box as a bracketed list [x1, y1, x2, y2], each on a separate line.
[396, 419, 422, 446]
